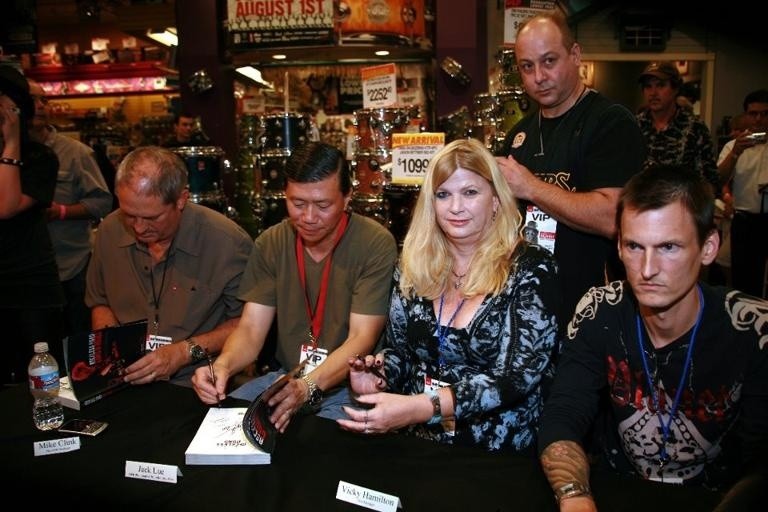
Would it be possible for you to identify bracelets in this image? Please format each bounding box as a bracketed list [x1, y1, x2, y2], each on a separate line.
[58, 201, 64, 221]
[2, 155, 25, 170]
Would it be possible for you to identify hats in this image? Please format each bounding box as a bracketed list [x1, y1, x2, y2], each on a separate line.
[634, 61, 684, 88]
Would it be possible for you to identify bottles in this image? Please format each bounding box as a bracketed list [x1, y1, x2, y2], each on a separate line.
[29, 343, 64, 431]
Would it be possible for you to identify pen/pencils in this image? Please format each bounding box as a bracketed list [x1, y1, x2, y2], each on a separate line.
[355, 352, 407, 396]
[97, 324, 117, 331]
[205, 353, 226, 409]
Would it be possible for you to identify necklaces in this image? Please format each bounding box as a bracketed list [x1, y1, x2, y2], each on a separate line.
[447, 267, 474, 291]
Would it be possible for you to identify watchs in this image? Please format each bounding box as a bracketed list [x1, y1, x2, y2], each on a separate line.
[552, 480, 592, 498]
[731, 153, 738, 160]
[423, 389, 442, 425]
[184, 338, 206, 363]
[302, 376, 320, 404]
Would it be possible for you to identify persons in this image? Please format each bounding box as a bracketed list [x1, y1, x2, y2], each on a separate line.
[1, 61, 58, 380]
[161, 112, 205, 146]
[675, 97, 690, 110]
[630, 61, 717, 185]
[719, 114, 747, 217]
[25, 83, 110, 335]
[84, 146, 255, 385]
[538, 162, 768, 512]
[335, 140, 561, 454]
[716, 91, 768, 296]
[191, 142, 396, 433]
[490, 11, 647, 310]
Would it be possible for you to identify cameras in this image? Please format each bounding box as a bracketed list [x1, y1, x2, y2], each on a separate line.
[746, 132, 768, 144]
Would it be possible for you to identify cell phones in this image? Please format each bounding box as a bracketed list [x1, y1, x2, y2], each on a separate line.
[57, 418, 109, 437]
